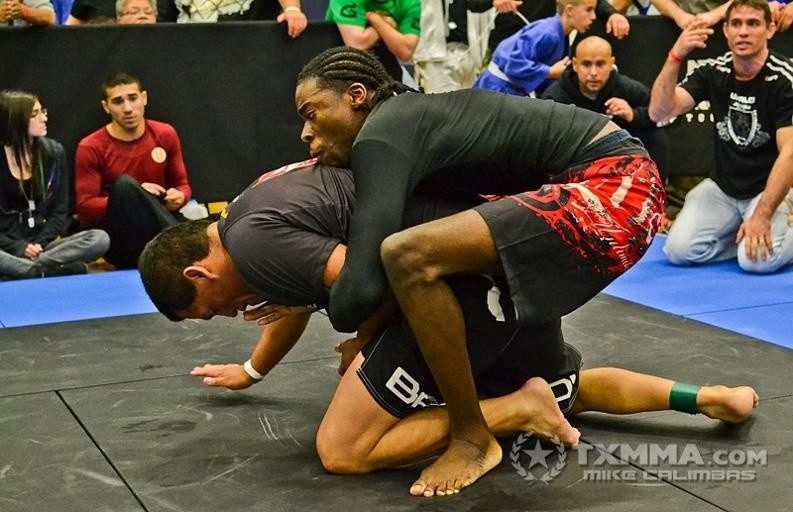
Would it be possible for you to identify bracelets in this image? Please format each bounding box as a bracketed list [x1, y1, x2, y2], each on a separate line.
[244, 359, 265, 380]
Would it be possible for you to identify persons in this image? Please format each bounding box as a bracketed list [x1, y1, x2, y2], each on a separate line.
[138, 157, 759, 474]
[0, 0, 792, 282]
[295, 47, 667, 498]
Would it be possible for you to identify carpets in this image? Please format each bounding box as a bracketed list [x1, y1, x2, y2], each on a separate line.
[601, 234, 792, 351]
[1, 268, 160, 331]
